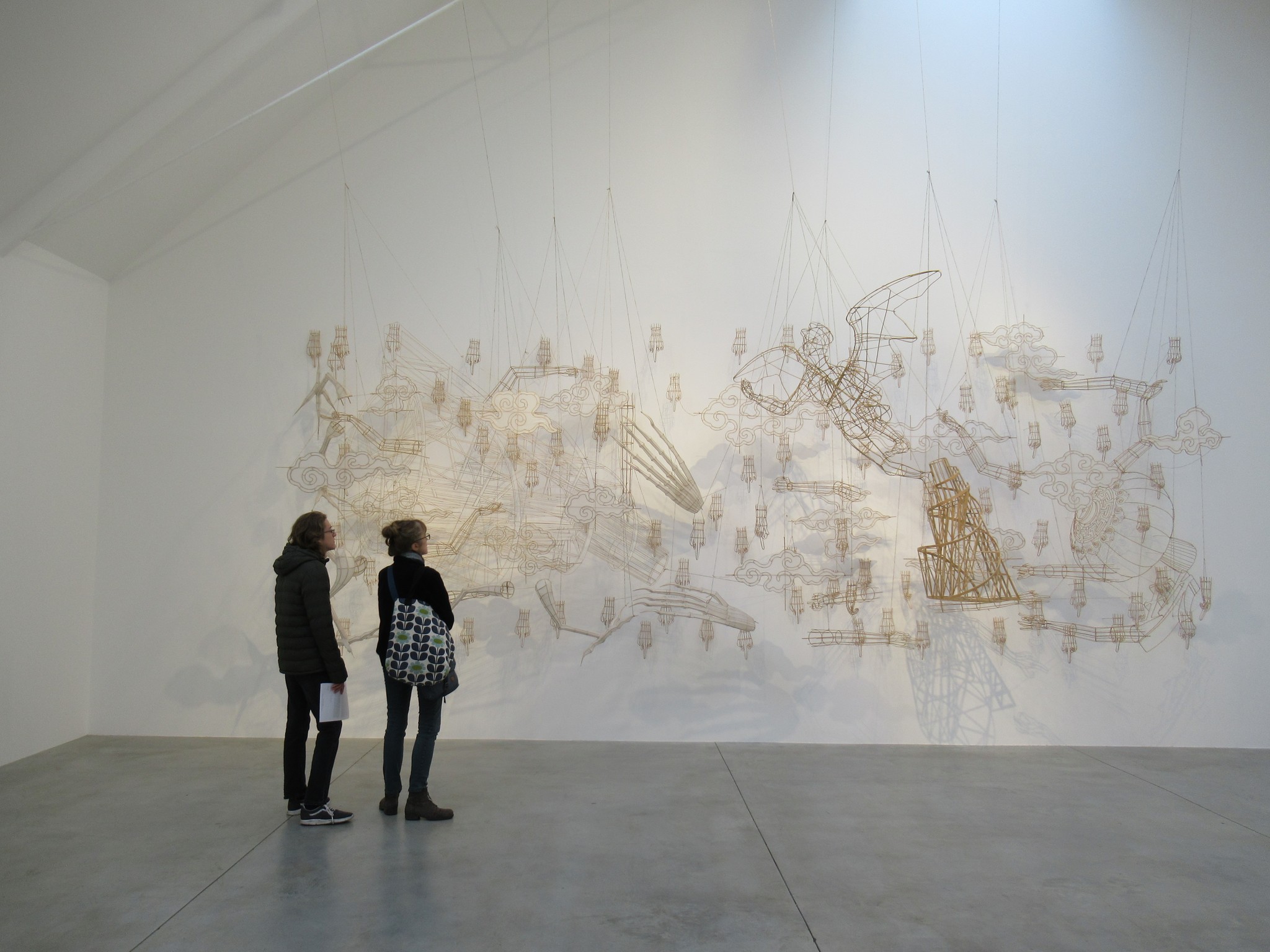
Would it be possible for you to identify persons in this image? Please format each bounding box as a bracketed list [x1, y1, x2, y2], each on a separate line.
[273, 511, 354, 825]
[376, 520, 459, 820]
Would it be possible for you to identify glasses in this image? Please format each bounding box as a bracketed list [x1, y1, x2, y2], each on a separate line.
[417, 533, 431, 541]
[324, 529, 335, 534]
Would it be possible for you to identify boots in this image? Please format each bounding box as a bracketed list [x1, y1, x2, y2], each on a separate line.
[404, 788, 454, 820]
[379, 789, 402, 815]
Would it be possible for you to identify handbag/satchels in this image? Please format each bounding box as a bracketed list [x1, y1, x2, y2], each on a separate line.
[384, 601, 456, 686]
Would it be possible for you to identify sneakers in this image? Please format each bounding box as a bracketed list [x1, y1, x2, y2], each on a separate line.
[299, 802, 354, 825]
[287, 795, 331, 815]
